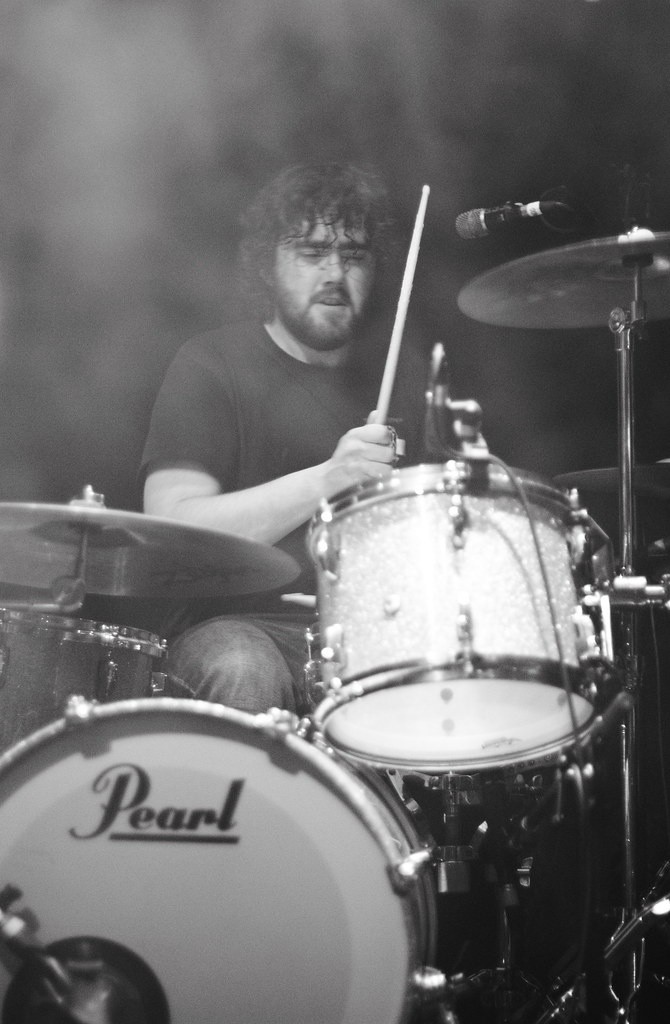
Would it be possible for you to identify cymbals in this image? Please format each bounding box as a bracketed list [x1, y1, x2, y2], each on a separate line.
[2, 496, 299, 603]
[552, 460, 670, 501]
[456, 232, 670, 329]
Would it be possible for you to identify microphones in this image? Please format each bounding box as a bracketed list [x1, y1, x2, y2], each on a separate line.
[454, 199, 557, 241]
[422, 340, 449, 455]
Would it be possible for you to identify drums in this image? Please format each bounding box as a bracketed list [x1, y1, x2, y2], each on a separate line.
[303, 457, 625, 769]
[0, 604, 168, 730]
[2, 695, 452, 1024]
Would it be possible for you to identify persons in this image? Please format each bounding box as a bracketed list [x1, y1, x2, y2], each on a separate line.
[136, 158, 432, 714]
